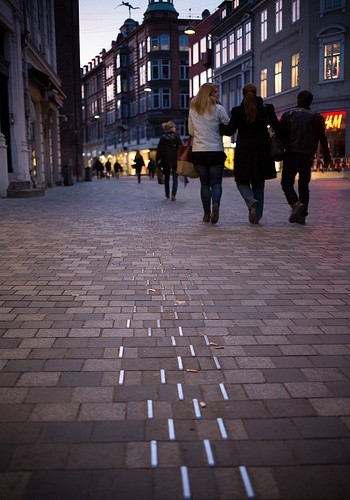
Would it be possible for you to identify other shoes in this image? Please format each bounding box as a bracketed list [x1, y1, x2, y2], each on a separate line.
[289, 201, 307, 225]
[211, 203, 219, 224]
[203, 214, 210, 223]
[249, 200, 261, 225]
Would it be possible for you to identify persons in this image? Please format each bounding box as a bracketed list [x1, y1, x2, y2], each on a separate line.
[114, 161, 121, 178]
[133, 151, 145, 184]
[279, 90, 331, 224]
[147, 158, 156, 179]
[219, 83, 282, 224]
[119, 1, 140, 17]
[156, 121, 183, 201]
[105, 160, 111, 177]
[188, 83, 230, 224]
[93, 160, 104, 179]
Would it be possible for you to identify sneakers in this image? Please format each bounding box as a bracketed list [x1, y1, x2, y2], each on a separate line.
[166, 194, 170, 199]
[171, 195, 176, 201]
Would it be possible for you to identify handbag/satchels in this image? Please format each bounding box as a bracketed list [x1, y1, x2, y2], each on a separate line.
[270, 135, 298, 164]
[176, 134, 199, 178]
